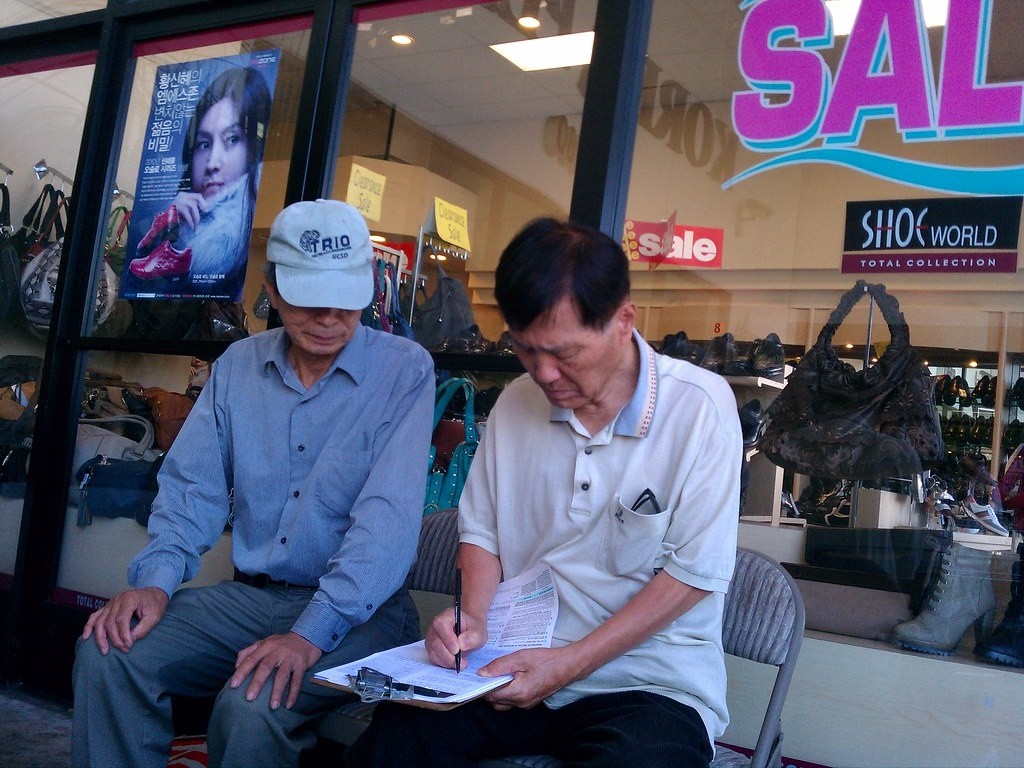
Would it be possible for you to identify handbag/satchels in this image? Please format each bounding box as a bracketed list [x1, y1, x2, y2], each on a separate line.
[1, 171, 248, 530]
[757, 280, 945, 480]
[425, 377, 481, 516]
[361, 241, 477, 346]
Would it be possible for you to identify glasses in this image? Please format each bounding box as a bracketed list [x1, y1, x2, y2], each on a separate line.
[631, 488, 661, 515]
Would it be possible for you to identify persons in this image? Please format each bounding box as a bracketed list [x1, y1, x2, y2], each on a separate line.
[190, 357, 209, 386]
[69, 199, 435, 768]
[993, 447, 1024, 536]
[348, 217, 743, 767]
[167, 66, 271, 291]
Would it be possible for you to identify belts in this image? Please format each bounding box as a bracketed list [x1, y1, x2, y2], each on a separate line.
[272, 578, 322, 592]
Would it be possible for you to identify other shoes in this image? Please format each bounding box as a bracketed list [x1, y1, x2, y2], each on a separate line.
[779, 470, 928, 527]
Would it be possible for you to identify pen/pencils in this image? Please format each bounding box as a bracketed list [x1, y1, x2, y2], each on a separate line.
[454, 568, 462, 676]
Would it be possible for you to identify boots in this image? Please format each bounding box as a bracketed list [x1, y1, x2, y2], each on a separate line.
[891, 534, 998, 656]
[971, 544, 1024, 670]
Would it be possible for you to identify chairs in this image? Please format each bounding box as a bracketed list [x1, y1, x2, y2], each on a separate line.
[316, 505, 460, 746]
[480, 548, 805, 768]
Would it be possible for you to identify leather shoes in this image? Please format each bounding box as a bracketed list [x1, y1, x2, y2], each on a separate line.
[698, 331, 737, 374]
[657, 330, 688, 356]
[739, 398, 770, 448]
[129, 240, 193, 283]
[724, 347, 752, 376]
[753, 332, 785, 378]
[429, 323, 516, 354]
[910, 365, 1024, 541]
[137, 204, 180, 257]
[686, 340, 706, 364]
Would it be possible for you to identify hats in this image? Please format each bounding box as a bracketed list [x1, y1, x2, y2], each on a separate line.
[265, 198, 375, 310]
